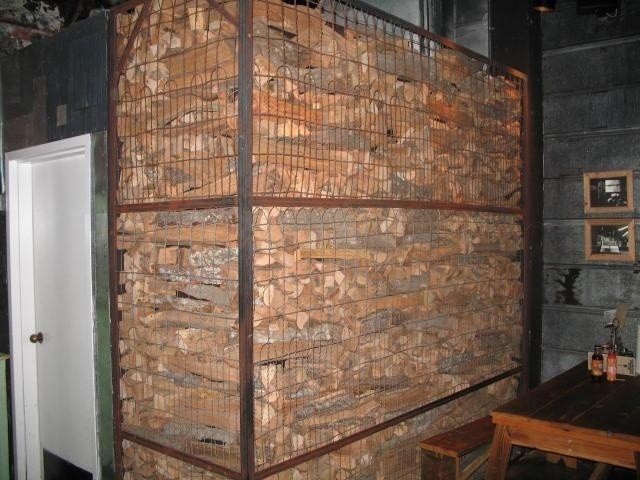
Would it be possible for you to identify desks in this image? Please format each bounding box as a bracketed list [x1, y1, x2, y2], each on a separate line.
[484, 359, 640, 480]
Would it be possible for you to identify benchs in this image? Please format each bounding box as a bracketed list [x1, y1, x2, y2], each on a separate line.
[419, 416, 495, 480]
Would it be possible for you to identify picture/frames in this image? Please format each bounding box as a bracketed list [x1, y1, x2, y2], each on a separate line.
[584, 218, 635, 263]
[583, 170, 633, 215]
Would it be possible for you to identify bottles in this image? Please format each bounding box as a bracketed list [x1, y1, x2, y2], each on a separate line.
[591, 345, 616, 381]
